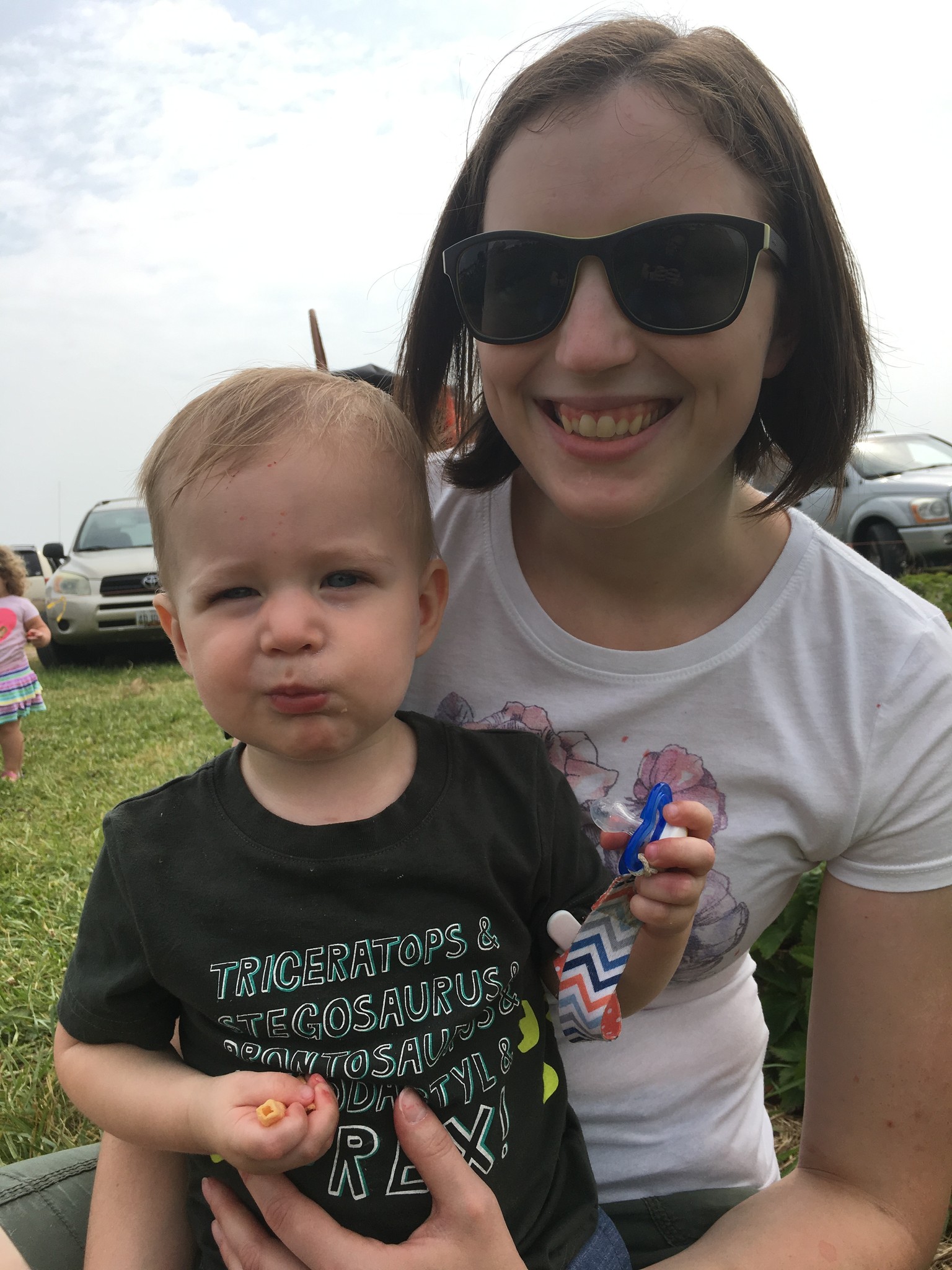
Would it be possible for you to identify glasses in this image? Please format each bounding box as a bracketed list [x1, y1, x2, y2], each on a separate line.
[441, 215, 788, 344]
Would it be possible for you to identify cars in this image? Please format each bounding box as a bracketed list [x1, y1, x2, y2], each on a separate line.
[750, 430, 952, 575]
[4, 545, 63, 617]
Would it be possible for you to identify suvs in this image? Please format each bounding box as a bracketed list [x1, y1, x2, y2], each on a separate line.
[43, 496, 180, 642]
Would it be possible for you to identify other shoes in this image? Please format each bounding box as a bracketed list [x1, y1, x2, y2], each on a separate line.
[2, 770, 24, 785]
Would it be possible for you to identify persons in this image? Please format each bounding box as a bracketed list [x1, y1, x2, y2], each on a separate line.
[51, 370, 714, 1270]
[0, 543, 53, 784]
[0, 1227, 31, 1270]
[80, 18, 952, 1270]
